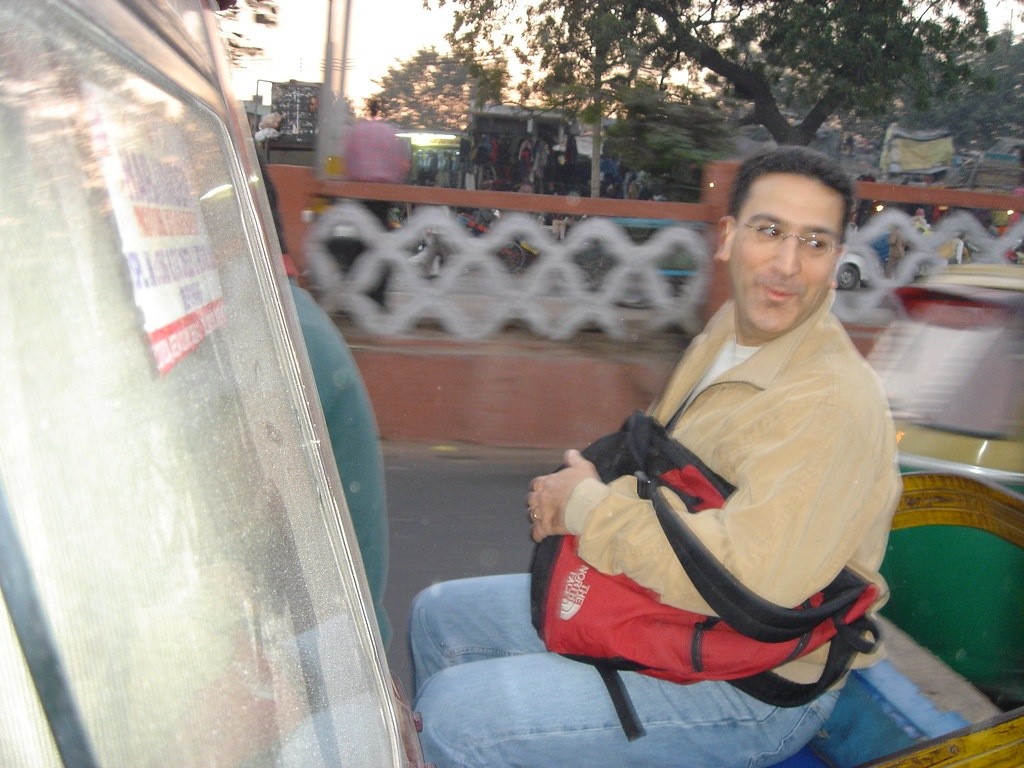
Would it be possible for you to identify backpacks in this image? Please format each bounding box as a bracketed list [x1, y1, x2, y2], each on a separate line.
[530, 407, 887, 747]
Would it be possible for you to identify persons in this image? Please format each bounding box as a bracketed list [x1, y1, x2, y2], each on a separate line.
[606, 174, 1024, 266]
[256, 156, 391, 655]
[405, 147, 903, 767]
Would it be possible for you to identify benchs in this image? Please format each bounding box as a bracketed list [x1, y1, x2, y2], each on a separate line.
[750, 464, 1024, 768]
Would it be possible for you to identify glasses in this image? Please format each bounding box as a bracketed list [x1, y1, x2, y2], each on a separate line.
[734, 220, 843, 261]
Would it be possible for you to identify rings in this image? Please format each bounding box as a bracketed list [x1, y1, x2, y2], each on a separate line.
[532, 506, 542, 519]
